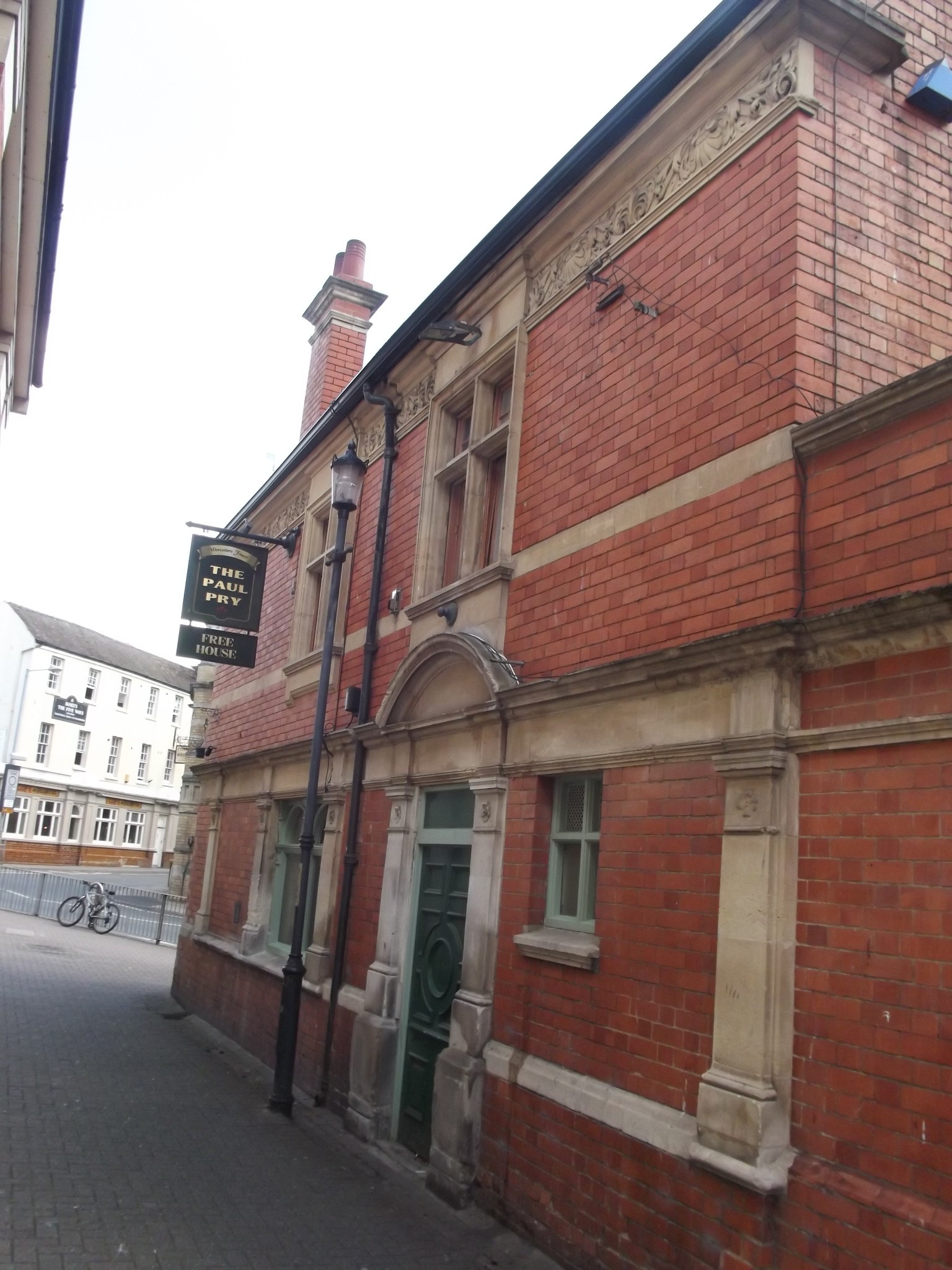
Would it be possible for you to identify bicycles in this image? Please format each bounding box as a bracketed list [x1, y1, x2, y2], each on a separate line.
[56, 879, 121, 934]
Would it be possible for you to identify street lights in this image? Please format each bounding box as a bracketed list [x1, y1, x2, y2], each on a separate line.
[264, 443, 372, 1115]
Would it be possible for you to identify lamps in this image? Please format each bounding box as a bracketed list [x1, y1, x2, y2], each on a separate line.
[419, 320, 482, 346]
